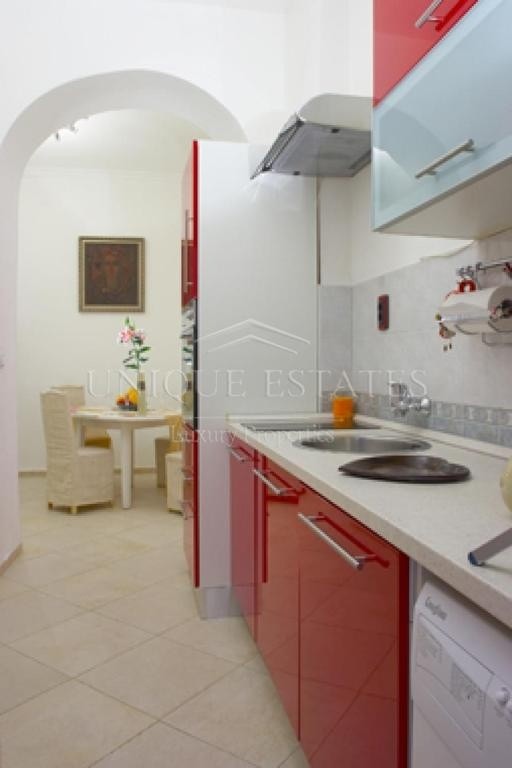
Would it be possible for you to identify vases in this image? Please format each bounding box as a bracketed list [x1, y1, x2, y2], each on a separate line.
[136, 372, 147, 416]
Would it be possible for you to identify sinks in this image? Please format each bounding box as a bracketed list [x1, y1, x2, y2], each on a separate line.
[295, 432, 432, 455]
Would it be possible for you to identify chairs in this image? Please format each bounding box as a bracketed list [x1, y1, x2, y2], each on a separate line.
[39, 385, 112, 514]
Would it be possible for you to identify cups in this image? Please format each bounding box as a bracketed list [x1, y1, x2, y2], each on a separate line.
[330, 395, 354, 428]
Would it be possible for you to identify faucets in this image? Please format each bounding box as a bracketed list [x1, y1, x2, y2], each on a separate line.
[390, 380, 433, 419]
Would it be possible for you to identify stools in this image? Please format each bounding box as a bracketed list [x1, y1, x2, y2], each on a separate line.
[153, 435, 183, 514]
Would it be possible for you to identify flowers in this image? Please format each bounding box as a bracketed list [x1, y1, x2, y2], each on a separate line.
[115, 317, 152, 374]
[117, 387, 138, 411]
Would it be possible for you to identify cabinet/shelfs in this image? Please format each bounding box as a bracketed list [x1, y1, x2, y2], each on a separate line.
[227, 431, 256, 641]
[298, 479, 410, 768]
[179, 137, 319, 592]
[371, 0, 511, 240]
[256, 454, 297, 742]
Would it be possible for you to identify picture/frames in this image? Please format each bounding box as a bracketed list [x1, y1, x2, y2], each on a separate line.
[76, 234, 146, 313]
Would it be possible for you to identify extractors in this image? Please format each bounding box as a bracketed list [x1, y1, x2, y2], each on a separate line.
[251, 92, 371, 180]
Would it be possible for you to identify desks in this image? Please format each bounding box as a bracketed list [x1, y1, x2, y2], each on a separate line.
[72, 406, 178, 510]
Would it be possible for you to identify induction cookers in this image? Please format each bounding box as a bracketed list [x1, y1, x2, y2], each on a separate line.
[243, 418, 379, 430]
